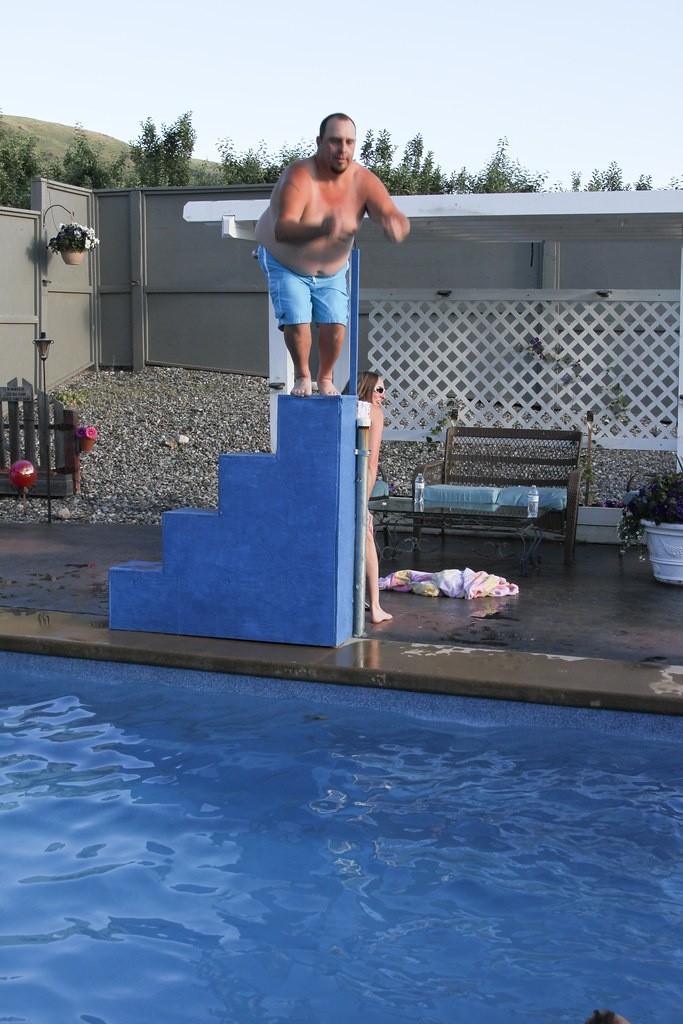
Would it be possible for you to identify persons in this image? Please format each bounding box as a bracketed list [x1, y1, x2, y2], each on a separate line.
[340, 372, 393, 623]
[252, 113, 411, 399]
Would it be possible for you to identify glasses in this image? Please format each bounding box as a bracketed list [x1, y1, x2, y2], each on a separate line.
[368, 384, 385, 394]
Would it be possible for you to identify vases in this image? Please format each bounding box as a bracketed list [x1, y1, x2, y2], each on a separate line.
[640, 519, 683, 586]
[80, 438, 94, 452]
[61, 250, 84, 265]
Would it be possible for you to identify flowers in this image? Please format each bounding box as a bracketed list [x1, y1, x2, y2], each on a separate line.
[76, 426, 97, 440]
[616, 473, 683, 564]
[46, 221, 100, 255]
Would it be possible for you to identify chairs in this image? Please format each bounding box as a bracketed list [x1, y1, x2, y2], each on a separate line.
[369, 465, 390, 553]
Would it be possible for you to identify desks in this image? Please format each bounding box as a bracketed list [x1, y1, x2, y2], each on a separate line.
[369, 498, 549, 578]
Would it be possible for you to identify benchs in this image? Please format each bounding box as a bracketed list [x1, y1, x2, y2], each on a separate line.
[410, 426, 588, 564]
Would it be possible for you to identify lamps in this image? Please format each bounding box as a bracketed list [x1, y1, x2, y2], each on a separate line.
[31, 330, 55, 361]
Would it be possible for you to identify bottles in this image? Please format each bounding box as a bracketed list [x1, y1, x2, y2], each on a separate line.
[528, 485, 539, 518]
[415, 473, 425, 503]
